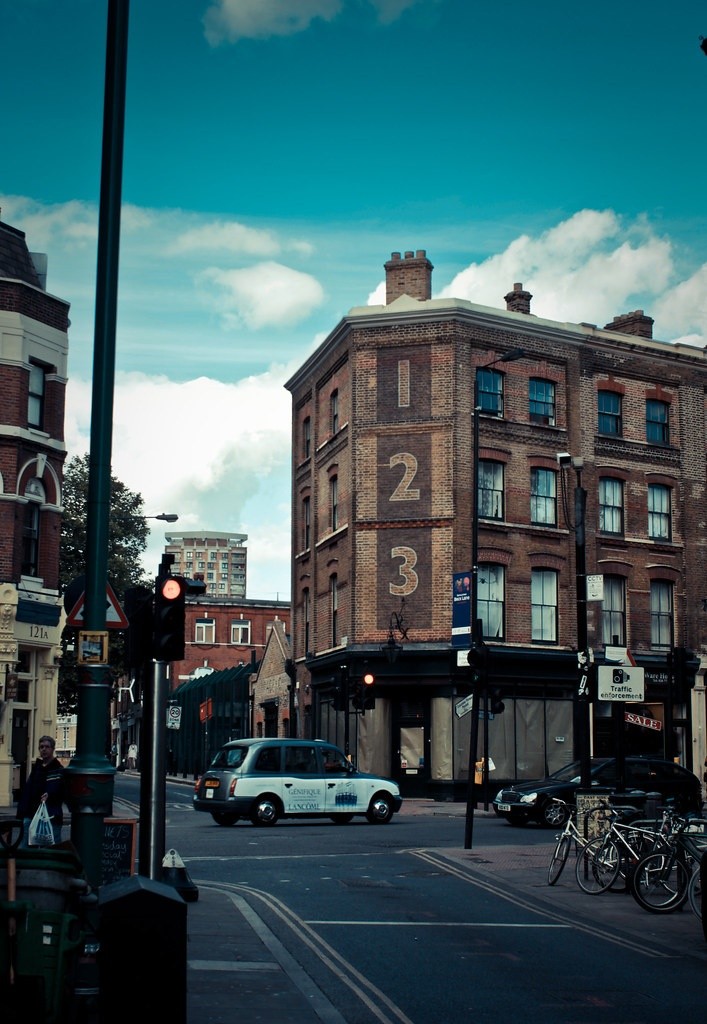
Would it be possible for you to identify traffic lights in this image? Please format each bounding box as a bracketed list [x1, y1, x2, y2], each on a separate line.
[491, 686, 505, 714]
[124, 576, 186, 661]
[672, 645, 700, 689]
[469, 647, 488, 674]
[361, 673, 375, 709]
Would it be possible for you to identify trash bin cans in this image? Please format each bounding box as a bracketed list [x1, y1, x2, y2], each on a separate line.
[573, 787, 616, 857]
[608, 790, 661, 889]
[99, 876, 188, 1024]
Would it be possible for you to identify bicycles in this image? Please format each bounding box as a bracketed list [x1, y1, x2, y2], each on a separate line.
[548, 797, 707, 923]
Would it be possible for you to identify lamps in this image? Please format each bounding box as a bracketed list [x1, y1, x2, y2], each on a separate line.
[383, 596, 410, 672]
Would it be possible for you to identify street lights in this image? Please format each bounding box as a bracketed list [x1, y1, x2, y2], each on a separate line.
[473, 349, 524, 645]
[557, 453, 591, 760]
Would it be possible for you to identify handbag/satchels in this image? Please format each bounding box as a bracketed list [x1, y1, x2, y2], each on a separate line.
[28, 800, 56, 846]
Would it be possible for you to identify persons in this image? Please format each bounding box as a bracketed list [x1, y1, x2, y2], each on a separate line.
[128, 740, 137, 769]
[17, 736, 65, 848]
[110, 741, 118, 767]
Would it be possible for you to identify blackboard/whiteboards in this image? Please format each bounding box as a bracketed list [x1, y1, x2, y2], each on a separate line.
[100, 818, 138, 885]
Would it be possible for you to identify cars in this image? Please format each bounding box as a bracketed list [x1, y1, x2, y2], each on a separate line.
[493, 755, 700, 828]
[192, 740, 403, 827]
